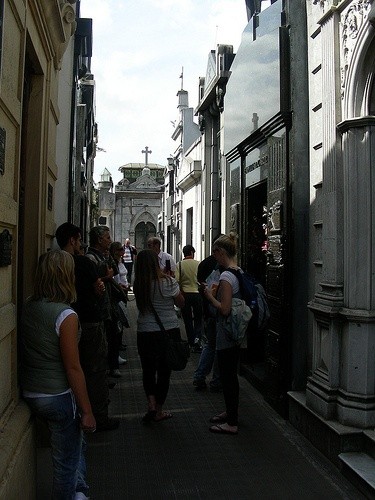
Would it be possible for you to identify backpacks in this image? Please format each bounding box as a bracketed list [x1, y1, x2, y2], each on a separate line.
[214, 268, 272, 343]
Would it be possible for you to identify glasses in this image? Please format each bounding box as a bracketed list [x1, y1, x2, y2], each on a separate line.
[209, 413, 227, 421]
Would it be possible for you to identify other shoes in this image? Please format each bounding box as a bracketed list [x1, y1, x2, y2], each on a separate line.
[141, 411, 157, 423]
[209, 381, 222, 393]
[193, 337, 204, 353]
[194, 381, 208, 391]
[118, 356, 127, 364]
[73, 491, 90, 500]
[103, 418, 121, 431]
[155, 412, 173, 421]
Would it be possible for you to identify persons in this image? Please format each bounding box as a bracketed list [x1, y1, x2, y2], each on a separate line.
[84, 226, 122, 379]
[146, 237, 176, 279]
[110, 241, 130, 307]
[133, 249, 185, 422]
[192, 234, 222, 394]
[203, 232, 248, 435]
[175, 245, 202, 353]
[18, 249, 96, 500]
[121, 238, 137, 291]
[54, 222, 120, 433]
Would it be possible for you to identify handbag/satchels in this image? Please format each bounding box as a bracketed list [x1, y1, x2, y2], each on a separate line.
[157, 331, 190, 371]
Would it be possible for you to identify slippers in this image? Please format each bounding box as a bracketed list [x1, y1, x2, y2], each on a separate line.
[209, 424, 240, 434]
